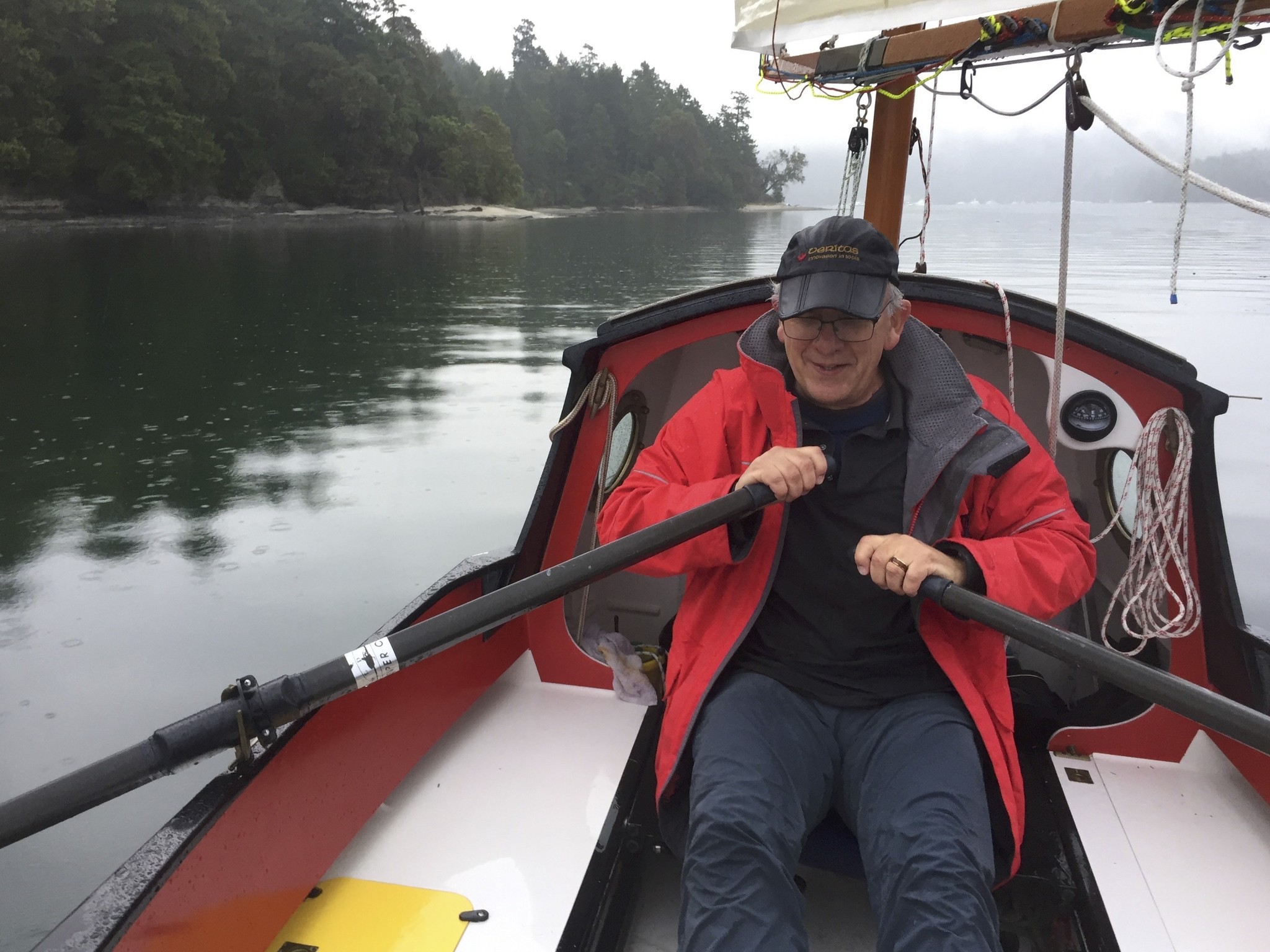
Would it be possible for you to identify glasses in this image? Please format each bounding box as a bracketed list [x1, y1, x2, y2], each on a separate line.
[780, 301, 892, 344]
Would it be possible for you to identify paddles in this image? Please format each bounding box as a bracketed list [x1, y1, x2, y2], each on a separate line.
[1, 450, 841, 853]
[917, 574, 1269, 757]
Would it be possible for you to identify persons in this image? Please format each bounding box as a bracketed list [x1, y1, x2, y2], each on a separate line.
[596, 217, 1095, 952]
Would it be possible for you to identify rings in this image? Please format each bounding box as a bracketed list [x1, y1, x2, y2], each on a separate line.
[890, 556, 909, 573]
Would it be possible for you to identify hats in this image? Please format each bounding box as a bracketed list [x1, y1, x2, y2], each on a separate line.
[775, 216, 900, 321]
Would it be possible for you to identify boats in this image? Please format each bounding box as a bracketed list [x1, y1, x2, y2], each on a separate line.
[32, 3, 1269, 952]
